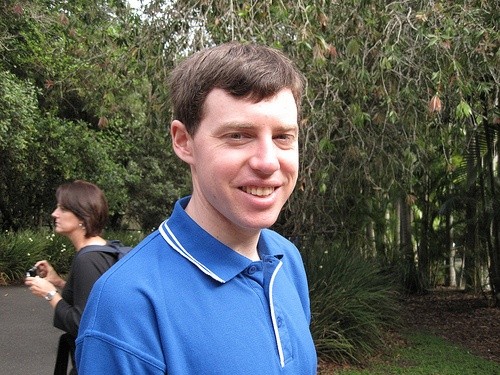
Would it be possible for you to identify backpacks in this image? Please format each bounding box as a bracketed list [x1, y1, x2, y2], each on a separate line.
[77, 240, 132, 261]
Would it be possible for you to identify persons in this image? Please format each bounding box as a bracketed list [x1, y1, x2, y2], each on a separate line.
[75, 40, 317, 375]
[24, 179, 121, 375]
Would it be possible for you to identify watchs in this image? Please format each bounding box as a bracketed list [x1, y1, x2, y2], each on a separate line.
[45, 289, 60, 303]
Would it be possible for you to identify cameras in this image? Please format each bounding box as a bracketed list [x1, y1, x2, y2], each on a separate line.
[26, 266, 39, 278]
